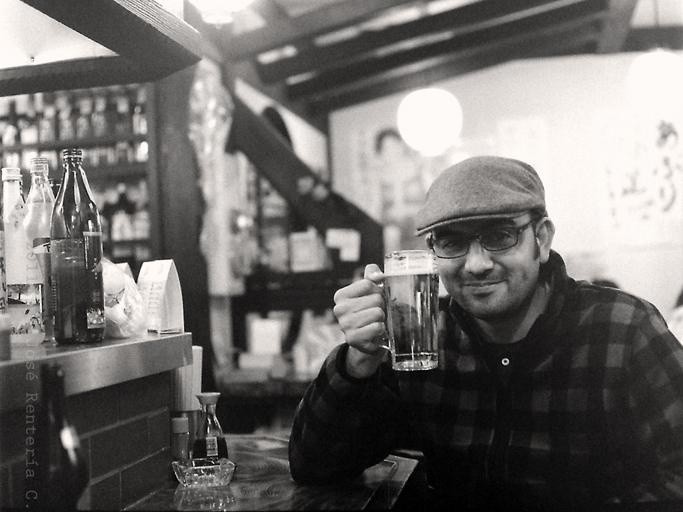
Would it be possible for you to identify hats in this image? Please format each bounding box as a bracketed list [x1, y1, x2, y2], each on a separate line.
[414, 157, 546, 237]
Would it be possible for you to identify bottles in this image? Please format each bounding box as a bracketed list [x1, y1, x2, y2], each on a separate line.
[1, 86, 155, 263]
[170, 416, 191, 461]
[15, 158, 55, 344]
[189, 392, 229, 462]
[0, 181, 10, 363]
[49, 145, 105, 343]
[1, 165, 25, 206]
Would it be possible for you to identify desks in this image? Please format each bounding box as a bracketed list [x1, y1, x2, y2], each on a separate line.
[117, 432, 426, 509]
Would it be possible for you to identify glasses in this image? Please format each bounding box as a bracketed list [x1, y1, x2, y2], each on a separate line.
[426, 217, 538, 259]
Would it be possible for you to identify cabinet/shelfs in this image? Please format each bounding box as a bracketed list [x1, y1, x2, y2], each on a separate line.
[0, 84, 149, 285]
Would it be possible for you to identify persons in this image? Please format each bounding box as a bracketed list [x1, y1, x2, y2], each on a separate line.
[287, 156, 681, 508]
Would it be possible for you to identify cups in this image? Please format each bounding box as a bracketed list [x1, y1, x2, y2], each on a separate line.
[367, 248, 442, 374]
